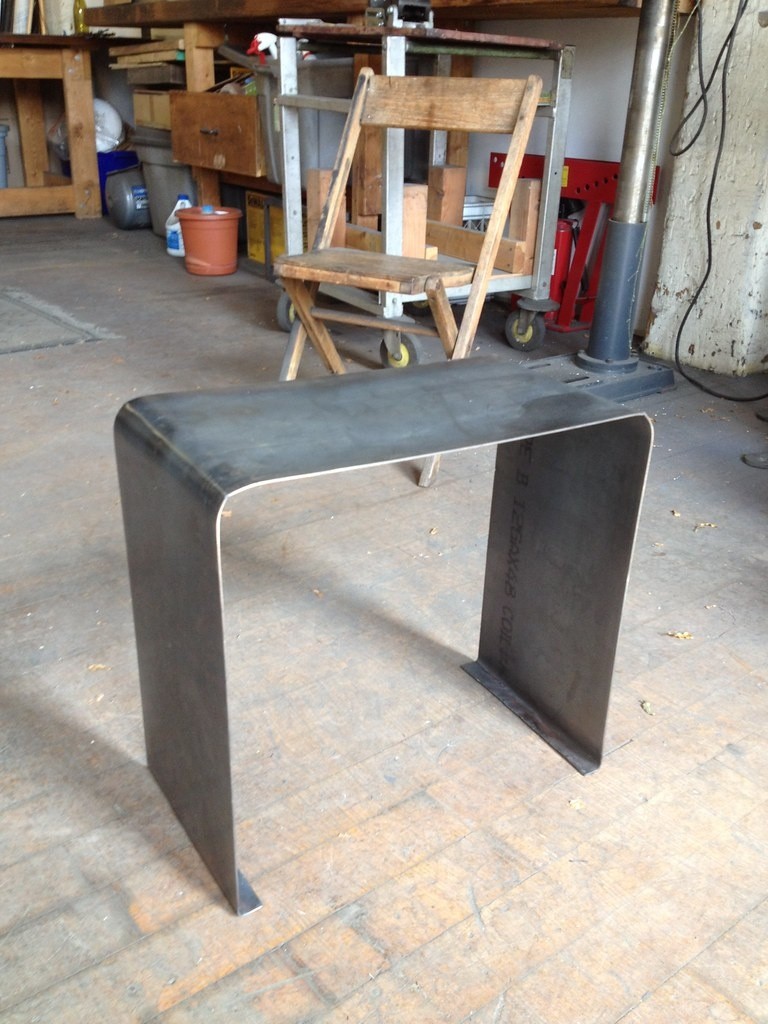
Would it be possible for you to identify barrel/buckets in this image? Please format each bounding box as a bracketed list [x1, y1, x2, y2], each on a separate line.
[175, 204, 242, 276]
[62, 150, 141, 215]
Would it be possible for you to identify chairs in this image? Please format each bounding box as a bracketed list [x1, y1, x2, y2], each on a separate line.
[270, 66, 542, 488]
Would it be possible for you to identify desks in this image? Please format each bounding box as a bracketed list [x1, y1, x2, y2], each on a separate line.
[1, 34, 105, 220]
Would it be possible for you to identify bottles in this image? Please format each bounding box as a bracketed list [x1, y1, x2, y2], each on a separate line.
[73, 0, 89, 36]
[165, 194, 192, 257]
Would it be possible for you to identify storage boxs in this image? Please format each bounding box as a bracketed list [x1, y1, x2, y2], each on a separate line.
[167, 77, 268, 180]
[254, 53, 357, 187]
